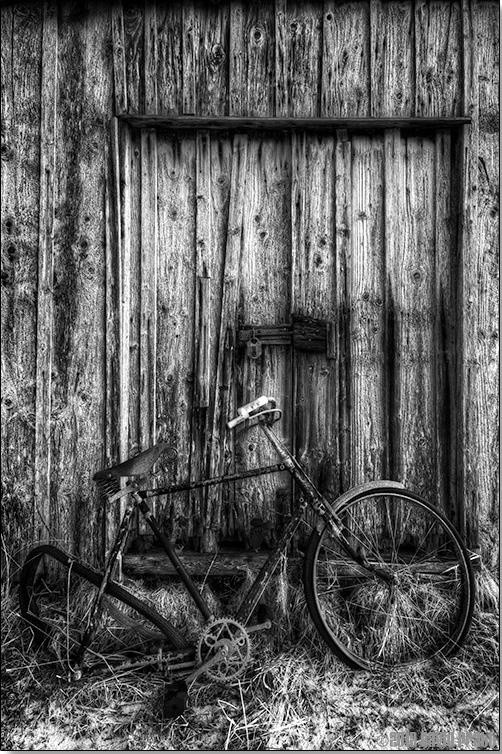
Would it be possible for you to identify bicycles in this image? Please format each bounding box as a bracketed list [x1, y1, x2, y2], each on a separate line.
[17, 395, 477, 708]
